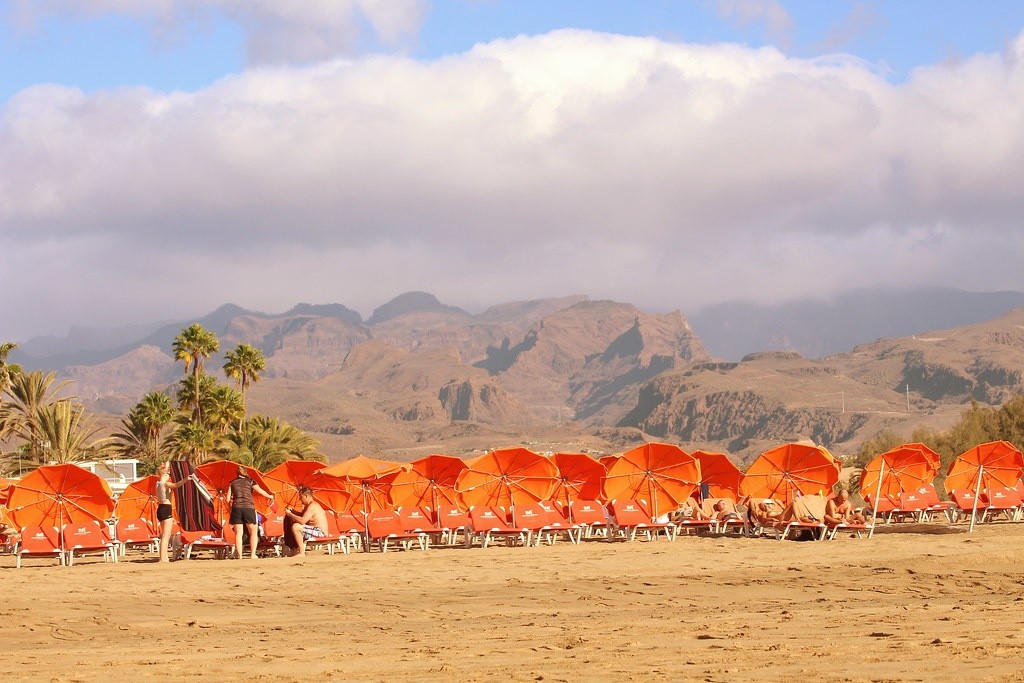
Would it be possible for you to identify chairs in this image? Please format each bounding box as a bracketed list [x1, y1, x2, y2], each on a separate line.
[0, 478, 1024, 569]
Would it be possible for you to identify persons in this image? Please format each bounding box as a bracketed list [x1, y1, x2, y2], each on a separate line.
[824, 490, 874, 527]
[156, 468, 193, 562]
[711, 500, 739, 521]
[225, 466, 273, 559]
[284, 487, 329, 558]
[671, 497, 711, 523]
[758, 502, 813, 524]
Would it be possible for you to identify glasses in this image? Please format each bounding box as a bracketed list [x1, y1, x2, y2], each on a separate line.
[166, 472, 171, 476]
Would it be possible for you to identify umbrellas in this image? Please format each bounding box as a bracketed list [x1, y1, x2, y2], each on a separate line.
[858, 443, 942, 537]
[0, 442, 841, 548]
[945, 439, 1024, 534]
